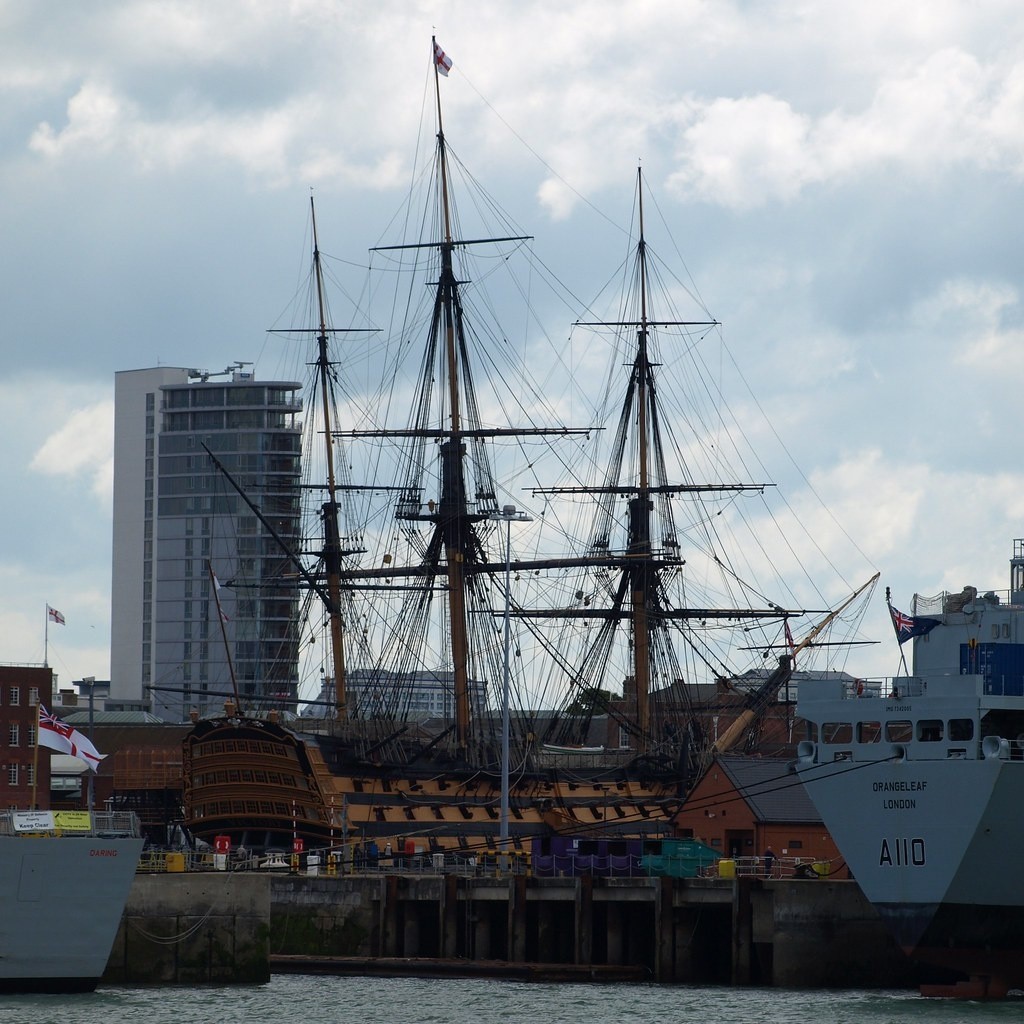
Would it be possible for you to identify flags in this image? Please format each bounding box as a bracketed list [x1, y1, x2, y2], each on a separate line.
[785, 621, 794, 658]
[434, 41, 450, 73]
[887, 604, 942, 646]
[49, 608, 64, 624]
[37, 702, 110, 775]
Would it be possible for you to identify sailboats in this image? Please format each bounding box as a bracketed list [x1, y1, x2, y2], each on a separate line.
[1, 27, 1024, 1003]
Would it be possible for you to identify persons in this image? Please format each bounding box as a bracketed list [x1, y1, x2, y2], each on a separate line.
[855, 679, 863, 696]
[961, 667, 967, 675]
[764, 845, 778, 879]
[353, 842, 393, 870]
[236, 845, 247, 860]
[889, 686, 898, 698]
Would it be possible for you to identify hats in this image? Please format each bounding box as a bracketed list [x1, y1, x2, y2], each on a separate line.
[386, 843, 391, 846]
